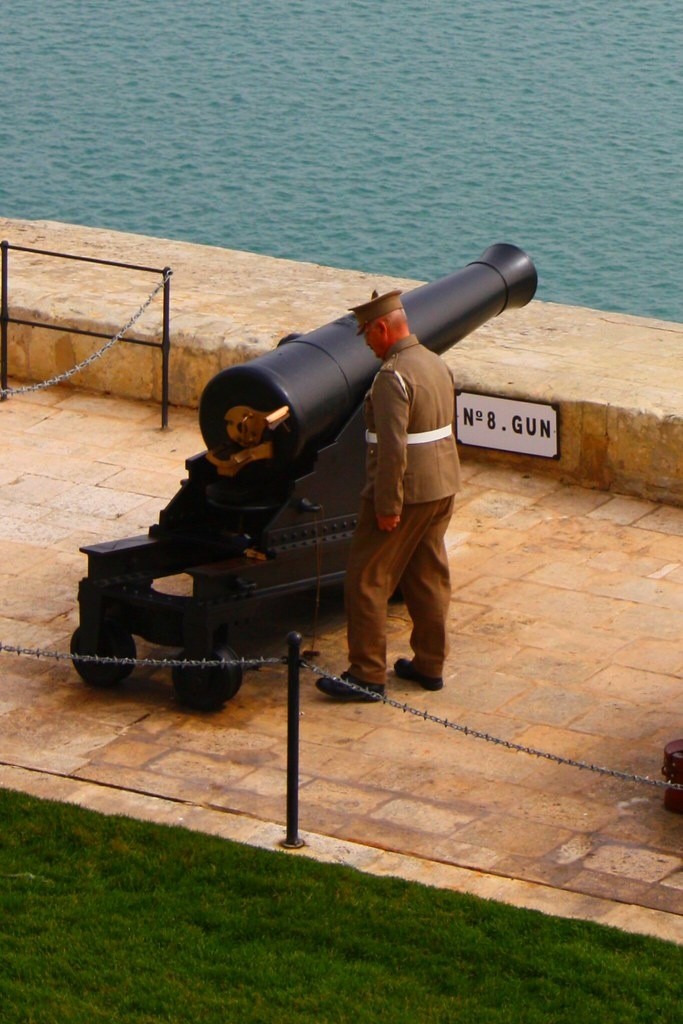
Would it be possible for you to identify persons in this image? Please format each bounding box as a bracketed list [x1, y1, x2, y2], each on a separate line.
[311, 286, 465, 704]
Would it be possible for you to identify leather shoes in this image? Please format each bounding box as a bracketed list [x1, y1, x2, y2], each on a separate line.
[315, 671, 385, 702]
[394, 657, 444, 690]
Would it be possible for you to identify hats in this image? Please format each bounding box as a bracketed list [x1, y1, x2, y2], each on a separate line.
[346, 289, 404, 337]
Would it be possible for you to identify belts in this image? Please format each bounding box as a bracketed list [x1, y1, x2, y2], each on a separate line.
[365, 424, 452, 445]
